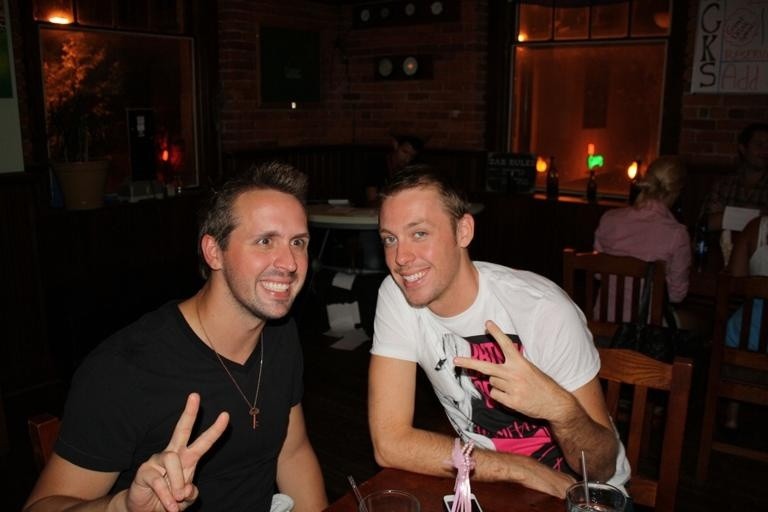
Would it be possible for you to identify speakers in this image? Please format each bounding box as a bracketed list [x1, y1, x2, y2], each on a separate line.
[352, 0, 461, 27]
[127, 108, 158, 181]
[375, 55, 435, 81]
[259, 25, 320, 103]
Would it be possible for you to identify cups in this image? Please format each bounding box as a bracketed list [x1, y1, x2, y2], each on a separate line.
[52, 159, 112, 213]
[564, 481, 625, 511]
[358, 489, 420, 512]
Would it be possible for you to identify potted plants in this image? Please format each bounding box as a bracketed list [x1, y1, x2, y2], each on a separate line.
[34, 92, 113, 212]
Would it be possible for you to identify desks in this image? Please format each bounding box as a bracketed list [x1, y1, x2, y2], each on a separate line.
[319, 465, 623, 511]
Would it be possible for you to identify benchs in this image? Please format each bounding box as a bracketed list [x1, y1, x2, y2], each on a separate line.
[221, 142, 484, 223]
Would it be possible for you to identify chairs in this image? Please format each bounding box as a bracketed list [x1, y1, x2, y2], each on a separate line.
[580, 345, 700, 508]
[555, 245, 690, 422]
[698, 270, 767, 504]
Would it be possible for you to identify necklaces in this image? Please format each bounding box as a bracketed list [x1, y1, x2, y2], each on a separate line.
[196, 290, 264, 429]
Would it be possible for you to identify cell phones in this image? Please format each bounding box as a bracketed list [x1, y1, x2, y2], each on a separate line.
[443, 493, 484, 512]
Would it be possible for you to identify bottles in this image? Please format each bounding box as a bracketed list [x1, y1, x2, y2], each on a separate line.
[628, 159, 641, 207]
[585, 170, 597, 201]
[546, 157, 559, 199]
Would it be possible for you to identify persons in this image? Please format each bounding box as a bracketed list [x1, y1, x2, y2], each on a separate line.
[723, 215, 768, 353]
[23, 160, 330, 512]
[696, 123, 768, 231]
[593, 156, 704, 416]
[367, 168, 658, 512]
